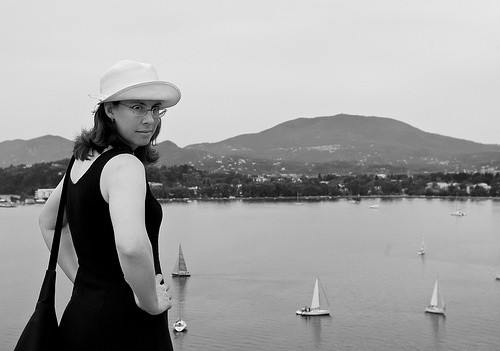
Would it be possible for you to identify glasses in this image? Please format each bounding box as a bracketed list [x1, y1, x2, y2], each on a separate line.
[119, 102, 167, 119]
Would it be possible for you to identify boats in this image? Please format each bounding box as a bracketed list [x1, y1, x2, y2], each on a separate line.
[172, 318, 190, 333]
[451, 211, 466, 217]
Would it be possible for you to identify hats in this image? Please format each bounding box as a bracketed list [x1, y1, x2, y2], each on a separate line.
[87, 60, 182, 115]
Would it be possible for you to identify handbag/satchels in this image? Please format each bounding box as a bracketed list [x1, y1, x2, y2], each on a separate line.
[14, 270, 61, 351]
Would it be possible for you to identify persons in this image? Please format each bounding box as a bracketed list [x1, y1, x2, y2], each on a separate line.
[38, 59, 173, 351]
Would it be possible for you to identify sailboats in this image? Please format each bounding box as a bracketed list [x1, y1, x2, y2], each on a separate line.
[418, 240, 427, 256]
[171, 244, 191, 279]
[294, 277, 332, 317]
[422, 280, 448, 316]
